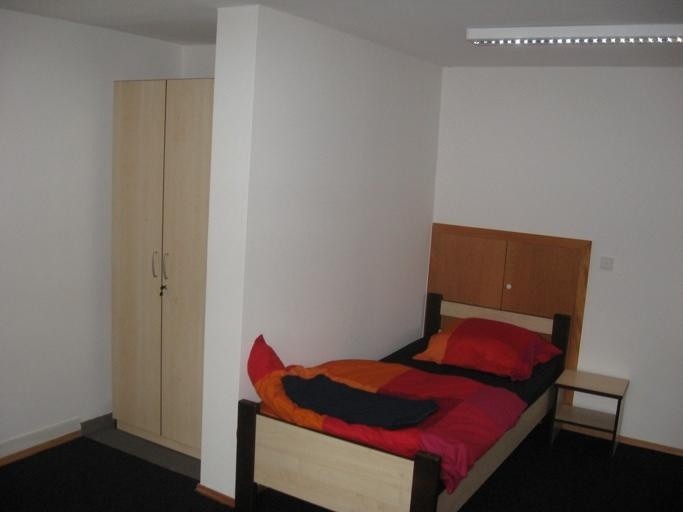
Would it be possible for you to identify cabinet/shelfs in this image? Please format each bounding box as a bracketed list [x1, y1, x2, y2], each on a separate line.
[111, 77, 215, 459]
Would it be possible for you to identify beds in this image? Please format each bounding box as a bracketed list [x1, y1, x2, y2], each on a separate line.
[237, 223, 594, 512]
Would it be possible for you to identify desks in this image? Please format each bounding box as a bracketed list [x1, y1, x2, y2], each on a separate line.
[550, 368, 630, 458]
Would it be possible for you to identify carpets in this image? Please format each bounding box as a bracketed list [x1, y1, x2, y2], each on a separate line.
[1, 423, 683, 512]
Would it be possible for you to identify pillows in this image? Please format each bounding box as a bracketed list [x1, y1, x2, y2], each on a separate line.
[412, 318, 563, 382]
